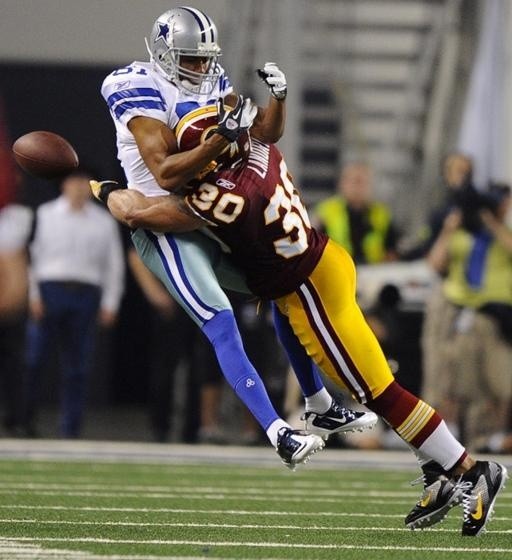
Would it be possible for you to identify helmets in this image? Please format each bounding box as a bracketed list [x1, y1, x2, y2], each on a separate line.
[150, 6, 222, 57]
[177, 104, 253, 179]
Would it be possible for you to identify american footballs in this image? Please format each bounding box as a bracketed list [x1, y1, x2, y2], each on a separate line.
[12, 131, 80, 181]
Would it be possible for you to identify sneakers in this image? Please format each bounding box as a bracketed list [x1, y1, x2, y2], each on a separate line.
[405, 460, 509, 536]
[275, 399, 379, 471]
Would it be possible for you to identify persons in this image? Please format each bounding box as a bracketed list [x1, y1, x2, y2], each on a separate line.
[89, 104, 507, 537]
[309, 154, 511, 452]
[100, 6, 378, 471]
[0, 152, 289, 446]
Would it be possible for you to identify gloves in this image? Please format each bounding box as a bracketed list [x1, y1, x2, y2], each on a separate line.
[216, 95, 258, 141]
[89, 179, 118, 203]
[255, 63, 288, 102]
